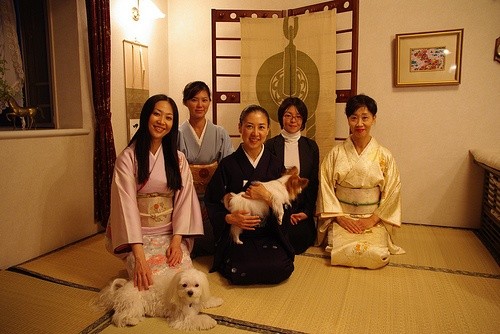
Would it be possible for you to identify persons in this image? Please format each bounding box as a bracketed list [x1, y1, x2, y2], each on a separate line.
[265, 97, 320, 255]
[321, 95, 406, 269]
[203, 105, 296, 285]
[104, 95, 204, 292]
[178, 81, 235, 256]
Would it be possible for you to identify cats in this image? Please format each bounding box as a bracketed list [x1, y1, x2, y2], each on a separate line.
[223, 165, 309, 245]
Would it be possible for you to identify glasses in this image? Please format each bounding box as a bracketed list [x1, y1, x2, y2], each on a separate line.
[284, 114, 303, 120]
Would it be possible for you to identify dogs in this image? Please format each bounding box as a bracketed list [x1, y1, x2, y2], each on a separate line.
[87, 264, 227, 334]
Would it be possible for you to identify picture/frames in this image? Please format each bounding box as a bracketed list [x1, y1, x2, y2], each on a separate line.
[394, 28, 464, 88]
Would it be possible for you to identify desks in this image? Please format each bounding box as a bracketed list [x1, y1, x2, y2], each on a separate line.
[472, 160, 500, 267]
